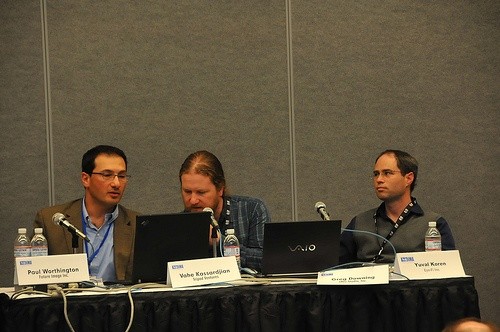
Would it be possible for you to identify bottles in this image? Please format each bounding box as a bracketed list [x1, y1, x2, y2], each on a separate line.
[30, 228, 49, 256]
[13, 228, 30, 258]
[222, 228, 241, 274]
[424, 221, 442, 252]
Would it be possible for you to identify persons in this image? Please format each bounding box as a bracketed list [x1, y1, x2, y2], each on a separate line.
[441, 317, 500, 332]
[30, 144, 141, 283]
[339, 149, 455, 263]
[177, 150, 271, 271]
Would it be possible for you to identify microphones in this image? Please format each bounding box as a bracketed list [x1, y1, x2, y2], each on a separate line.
[315, 201, 330, 221]
[52, 212, 90, 242]
[202, 207, 220, 231]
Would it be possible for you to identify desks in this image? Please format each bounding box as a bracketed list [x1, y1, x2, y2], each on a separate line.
[0, 272, 481, 332]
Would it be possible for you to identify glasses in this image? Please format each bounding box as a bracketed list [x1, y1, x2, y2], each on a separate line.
[92, 170, 132, 182]
[372, 170, 400, 178]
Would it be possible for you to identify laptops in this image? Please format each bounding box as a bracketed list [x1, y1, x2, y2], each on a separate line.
[102, 211, 212, 285]
[241, 219, 343, 277]
